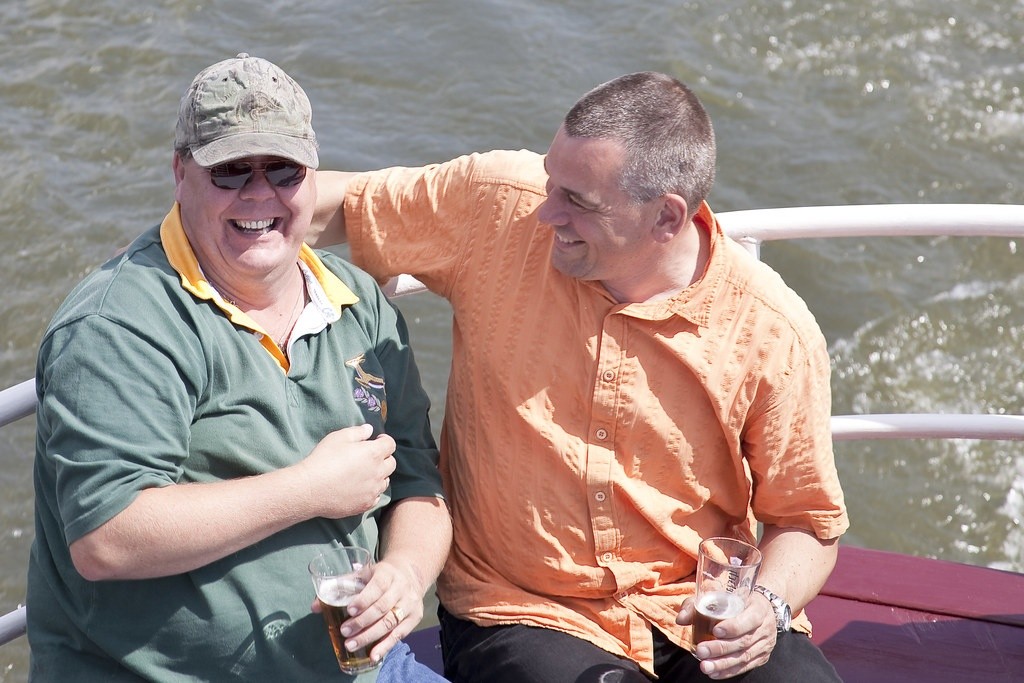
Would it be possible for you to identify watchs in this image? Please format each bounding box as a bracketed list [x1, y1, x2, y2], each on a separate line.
[754, 585, 791, 634]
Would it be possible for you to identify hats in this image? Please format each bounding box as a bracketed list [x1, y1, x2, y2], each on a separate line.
[175, 53, 321, 169]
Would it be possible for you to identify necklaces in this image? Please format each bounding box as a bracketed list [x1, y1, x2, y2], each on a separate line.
[204, 273, 303, 359]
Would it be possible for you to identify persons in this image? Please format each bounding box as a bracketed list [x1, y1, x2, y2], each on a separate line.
[25, 53, 454, 683]
[315, 73, 850, 683]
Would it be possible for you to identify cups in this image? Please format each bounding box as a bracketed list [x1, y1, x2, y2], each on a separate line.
[308, 546, 384, 675]
[691, 537, 762, 661]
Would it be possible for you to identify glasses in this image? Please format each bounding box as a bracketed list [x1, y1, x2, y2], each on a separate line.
[211, 161, 307, 190]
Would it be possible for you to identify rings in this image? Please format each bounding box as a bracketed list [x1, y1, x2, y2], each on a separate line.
[382, 479, 389, 492]
[392, 608, 406, 622]
